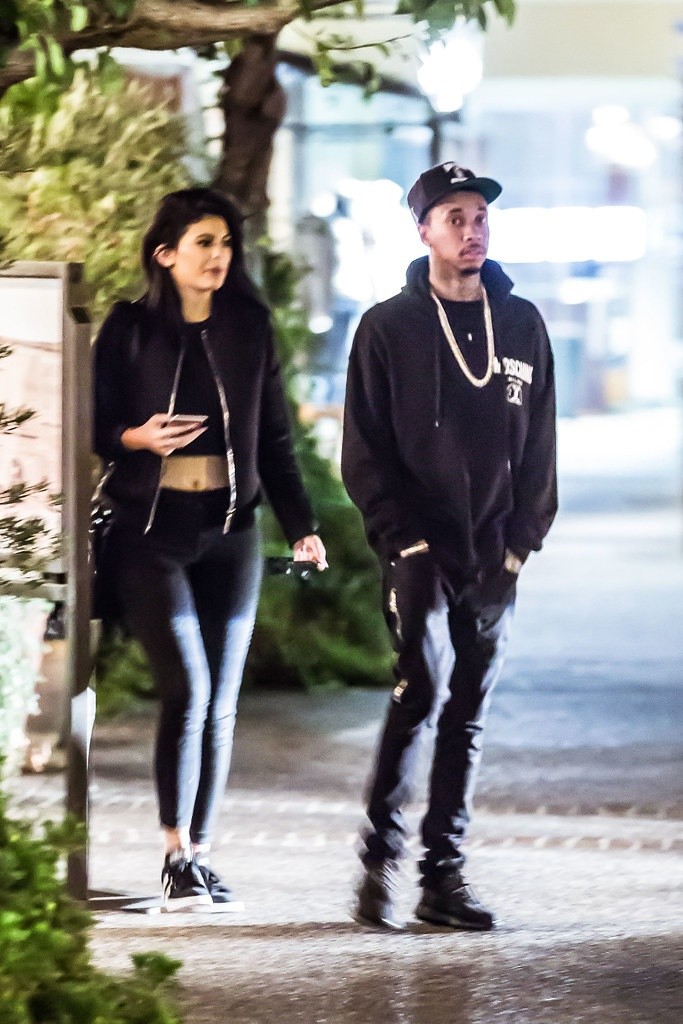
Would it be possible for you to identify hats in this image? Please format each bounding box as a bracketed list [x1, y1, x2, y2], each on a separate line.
[408, 161, 504, 222]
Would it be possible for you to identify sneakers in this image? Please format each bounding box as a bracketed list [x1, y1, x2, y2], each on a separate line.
[340, 859, 411, 929]
[197, 856, 243, 912]
[160, 855, 214, 913]
[413, 874, 499, 936]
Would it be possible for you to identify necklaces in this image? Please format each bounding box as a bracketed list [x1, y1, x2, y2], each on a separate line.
[430, 282, 494, 388]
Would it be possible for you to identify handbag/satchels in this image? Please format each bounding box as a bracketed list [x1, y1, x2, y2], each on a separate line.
[89, 497, 120, 619]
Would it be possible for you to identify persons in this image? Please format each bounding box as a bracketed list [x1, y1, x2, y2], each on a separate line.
[340, 160, 559, 930]
[89, 188, 329, 913]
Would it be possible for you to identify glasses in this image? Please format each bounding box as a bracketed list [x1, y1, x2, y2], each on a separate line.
[267, 555, 318, 580]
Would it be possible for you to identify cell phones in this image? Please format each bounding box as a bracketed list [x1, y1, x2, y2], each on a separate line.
[166, 414, 210, 436]
